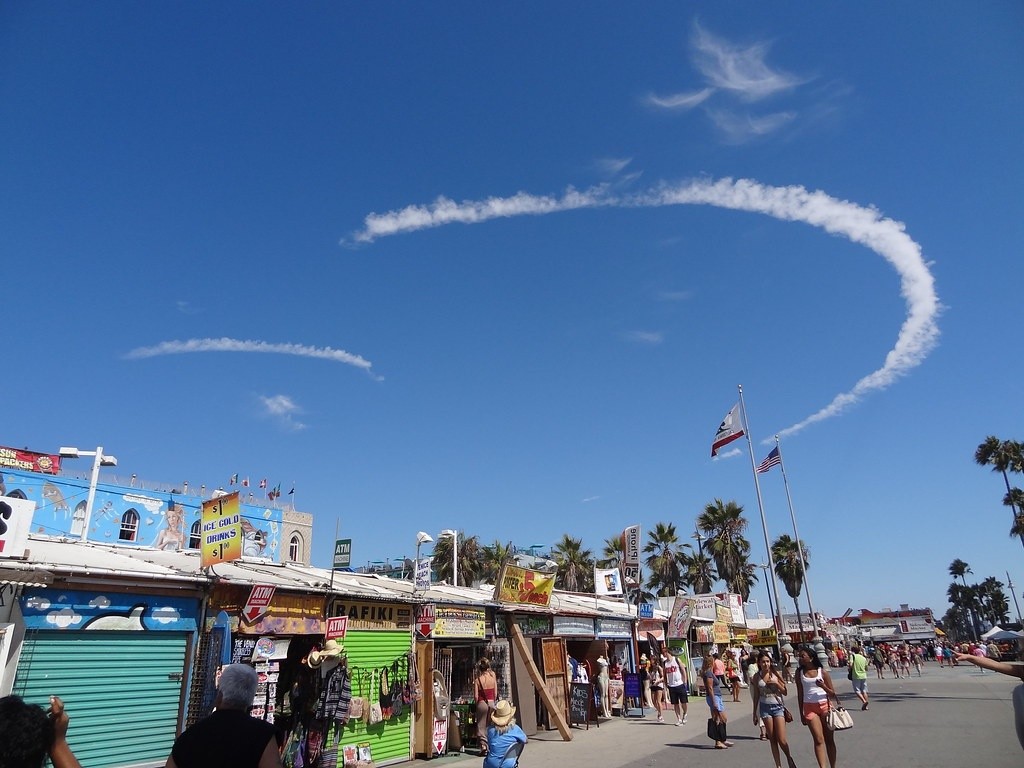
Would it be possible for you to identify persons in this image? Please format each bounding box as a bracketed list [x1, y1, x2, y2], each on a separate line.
[738, 650, 750, 688]
[916, 640, 962, 668]
[948, 648, 1024, 680]
[825, 646, 849, 667]
[712, 653, 733, 695]
[962, 638, 1002, 673]
[752, 651, 797, 768]
[779, 646, 795, 682]
[165, 663, 282, 768]
[700, 654, 734, 748]
[847, 645, 870, 711]
[0, 694, 82, 768]
[728, 652, 742, 703]
[647, 655, 666, 722]
[794, 646, 838, 768]
[859, 642, 924, 680]
[473, 659, 497, 756]
[747, 651, 768, 740]
[639, 655, 654, 708]
[596, 656, 612, 718]
[661, 648, 689, 726]
[483, 699, 529, 768]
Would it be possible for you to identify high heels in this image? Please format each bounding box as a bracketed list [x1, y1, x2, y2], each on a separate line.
[479, 749, 488, 756]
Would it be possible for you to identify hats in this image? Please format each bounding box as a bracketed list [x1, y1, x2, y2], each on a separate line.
[307, 651, 326, 670]
[491, 700, 516, 725]
[318, 639, 344, 656]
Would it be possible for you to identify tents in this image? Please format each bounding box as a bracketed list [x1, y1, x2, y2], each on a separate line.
[978, 626, 1024, 642]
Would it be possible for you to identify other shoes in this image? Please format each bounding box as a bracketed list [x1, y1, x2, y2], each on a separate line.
[861, 702, 868, 710]
[716, 744, 728, 749]
[658, 715, 664, 721]
[724, 741, 735, 747]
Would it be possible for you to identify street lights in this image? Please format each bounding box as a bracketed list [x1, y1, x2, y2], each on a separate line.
[1005, 571, 1023, 624]
[529, 544, 543, 555]
[983, 586, 998, 627]
[691, 522, 708, 594]
[759, 562, 778, 641]
[59, 447, 117, 543]
[437, 529, 458, 586]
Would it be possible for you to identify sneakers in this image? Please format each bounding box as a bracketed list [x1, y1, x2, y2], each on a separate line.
[676, 719, 682, 725]
[682, 713, 688, 723]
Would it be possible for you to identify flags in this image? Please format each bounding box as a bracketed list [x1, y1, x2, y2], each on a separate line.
[259, 478, 266, 489]
[288, 488, 294, 494]
[268, 484, 281, 501]
[710, 402, 745, 457]
[756, 446, 780, 474]
[229, 474, 238, 485]
[241, 480, 248, 487]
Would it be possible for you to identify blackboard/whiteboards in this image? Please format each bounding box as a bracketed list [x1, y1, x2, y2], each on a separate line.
[568, 682, 598, 723]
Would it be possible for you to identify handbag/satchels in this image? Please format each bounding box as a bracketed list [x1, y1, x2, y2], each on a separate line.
[825, 692, 854, 731]
[784, 709, 793, 723]
[707, 709, 726, 742]
[847, 654, 855, 680]
[488, 706, 495, 722]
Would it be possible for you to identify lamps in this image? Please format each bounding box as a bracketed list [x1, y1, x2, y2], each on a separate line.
[413, 531, 434, 593]
[623, 577, 635, 613]
[538, 560, 559, 570]
[677, 589, 687, 596]
